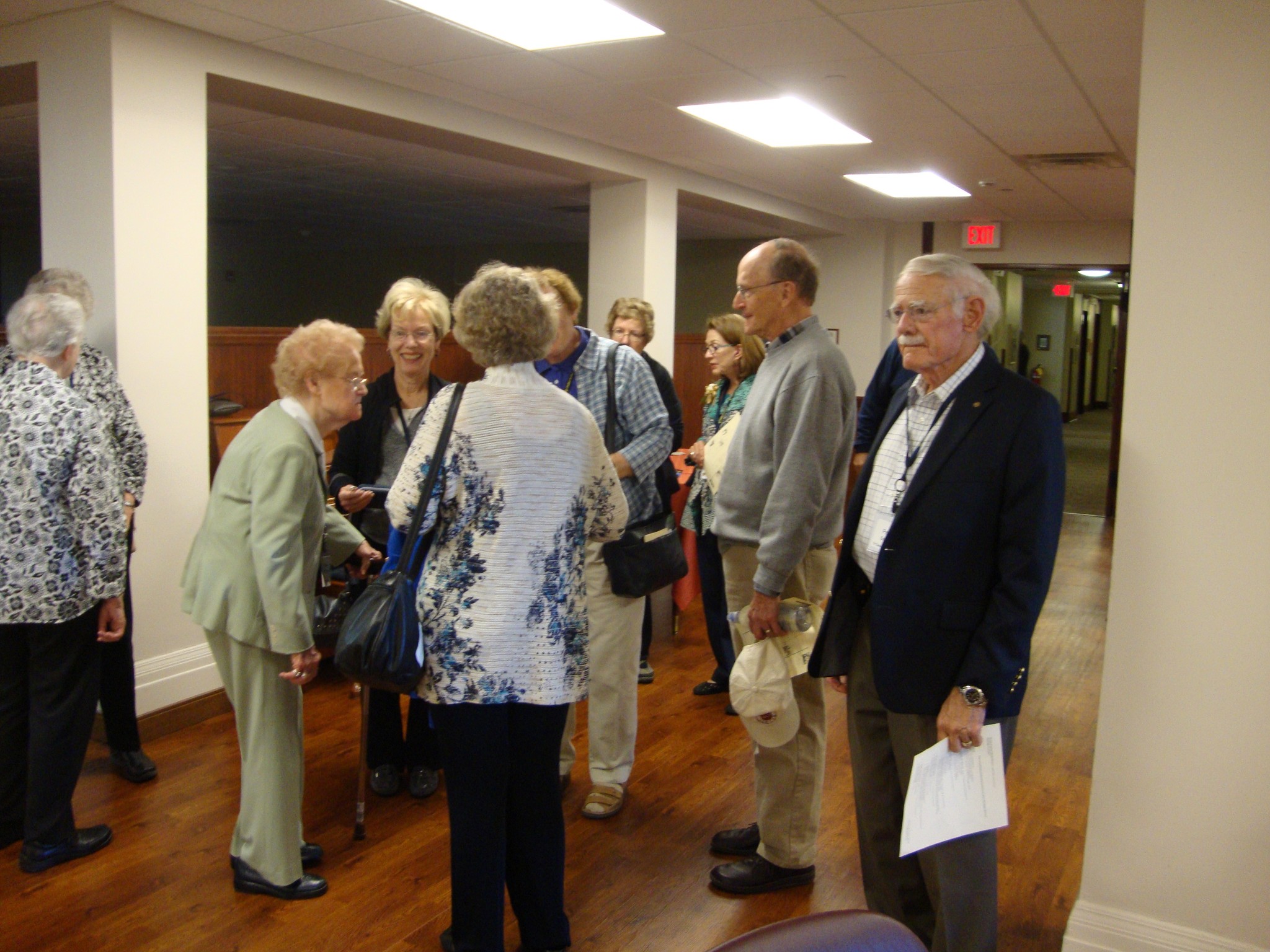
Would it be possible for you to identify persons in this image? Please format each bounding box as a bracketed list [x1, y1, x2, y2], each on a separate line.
[804, 251, 1070, 952]
[1017, 330, 1029, 377]
[323, 277, 456, 797]
[381, 262, 633, 952]
[704, 237, 859, 896]
[0, 266, 160, 787]
[602, 294, 686, 683]
[528, 267, 670, 823]
[0, 292, 129, 877]
[852, 333, 918, 471]
[688, 313, 766, 715]
[177, 316, 386, 901]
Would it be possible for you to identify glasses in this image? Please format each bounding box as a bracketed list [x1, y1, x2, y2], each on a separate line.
[332, 374, 369, 389]
[613, 328, 647, 341]
[887, 298, 967, 323]
[737, 279, 790, 298]
[390, 328, 437, 342]
[703, 344, 734, 354]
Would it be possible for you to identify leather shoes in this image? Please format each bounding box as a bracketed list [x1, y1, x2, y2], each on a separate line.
[710, 857, 817, 894]
[1, 828, 21, 849]
[710, 821, 758, 856]
[20, 824, 112, 872]
[234, 863, 329, 900]
[230, 844, 322, 868]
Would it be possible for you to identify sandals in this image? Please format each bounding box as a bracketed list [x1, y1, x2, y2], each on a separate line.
[581, 784, 626, 817]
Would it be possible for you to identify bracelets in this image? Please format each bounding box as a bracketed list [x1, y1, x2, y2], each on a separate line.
[122, 500, 135, 514]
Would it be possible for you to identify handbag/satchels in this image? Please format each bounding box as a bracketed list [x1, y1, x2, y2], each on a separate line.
[602, 510, 687, 597]
[315, 586, 352, 648]
[332, 570, 426, 695]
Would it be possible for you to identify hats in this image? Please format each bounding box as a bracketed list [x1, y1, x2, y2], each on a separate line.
[729, 637, 801, 747]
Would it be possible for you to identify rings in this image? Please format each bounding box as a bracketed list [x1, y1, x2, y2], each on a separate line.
[764, 629, 771, 633]
[689, 452, 695, 455]
[292, 668, 300, 677]
[961, 740, 972, 746]
[301, 672, 310, 678]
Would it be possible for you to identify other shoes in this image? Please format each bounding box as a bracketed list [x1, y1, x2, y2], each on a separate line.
[112, 748, 157, 781]
[435, 928, 456, 951]
[407, 764, 440, 798]
[372, 763, 402, 795]
[637, 659, 654, 682]
[692, 680, 725, 695]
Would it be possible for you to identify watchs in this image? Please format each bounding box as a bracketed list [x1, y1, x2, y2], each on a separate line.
[957, 685, 989, 707]
